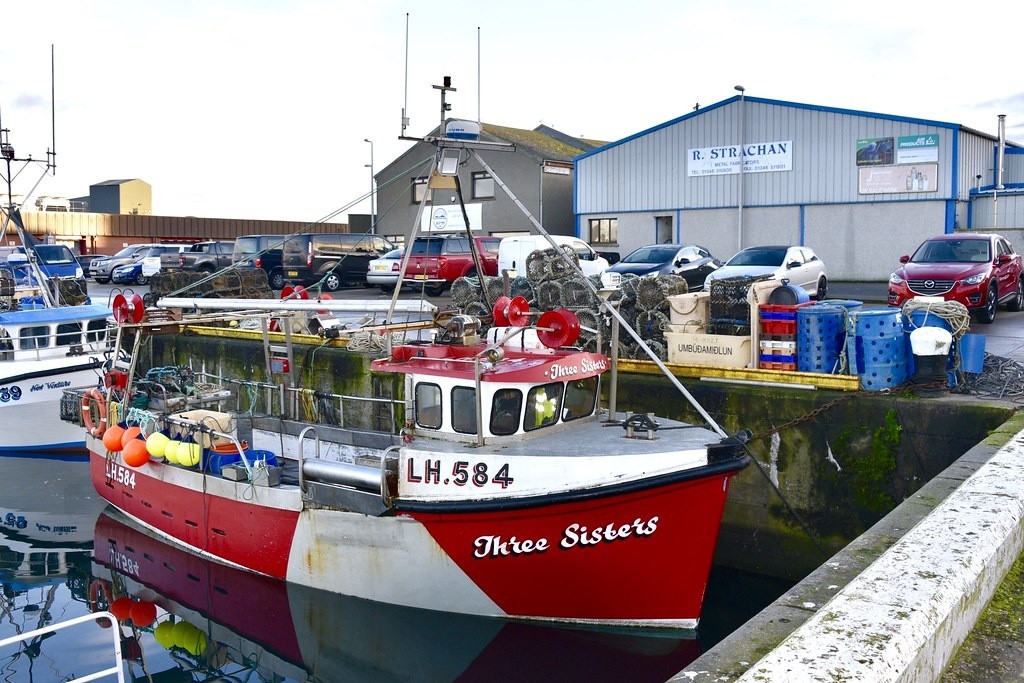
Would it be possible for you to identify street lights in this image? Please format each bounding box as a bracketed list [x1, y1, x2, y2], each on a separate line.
[363, 138, 376, 235]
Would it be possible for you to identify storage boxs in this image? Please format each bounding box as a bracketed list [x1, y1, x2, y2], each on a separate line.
[667, 292, 710, 333]
[758, 301, 818, 371]
[664, 331, 751, 369]
[169, 408, 231, 449]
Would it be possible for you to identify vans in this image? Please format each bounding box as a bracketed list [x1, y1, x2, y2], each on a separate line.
[401, 234, 496, 298]
[497, 237, 609, 278]
[0, 244, 89, 303]
[231, 233, 393, 291]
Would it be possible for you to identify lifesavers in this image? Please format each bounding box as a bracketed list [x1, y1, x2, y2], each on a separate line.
[82, 390, 106, 438]
[89, 579, 113, 629]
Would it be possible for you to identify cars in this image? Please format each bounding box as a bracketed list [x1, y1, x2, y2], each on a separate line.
[367, 251, 406, 291]
[602, 243, 717, 292]
[886, 231, 1024, 322]
[705, 246, 829, 305]
[77, 241, 231, 287]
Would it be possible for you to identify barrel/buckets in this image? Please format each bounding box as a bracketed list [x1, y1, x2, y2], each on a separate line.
[209, 440, 250, 477]
[769, 279, 809, 305]
[239, 449, 281, 485]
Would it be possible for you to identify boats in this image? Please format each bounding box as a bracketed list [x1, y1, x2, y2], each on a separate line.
[88, 500, 695, 683]
[0, 44, 133, 453]
[78, 10, 752, 640]
[0, 444, 110, 683]
